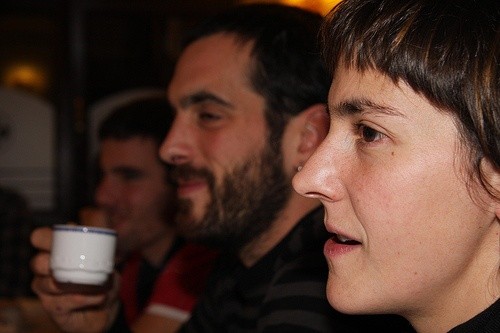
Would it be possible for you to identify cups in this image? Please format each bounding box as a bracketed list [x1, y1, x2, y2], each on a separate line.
[50, 224, 117, 285]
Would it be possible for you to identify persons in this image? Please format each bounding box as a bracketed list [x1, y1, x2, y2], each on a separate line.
[94, 99, 217, 333]
[290, 0, 500, 333]
[30, 4, 333, 333]
